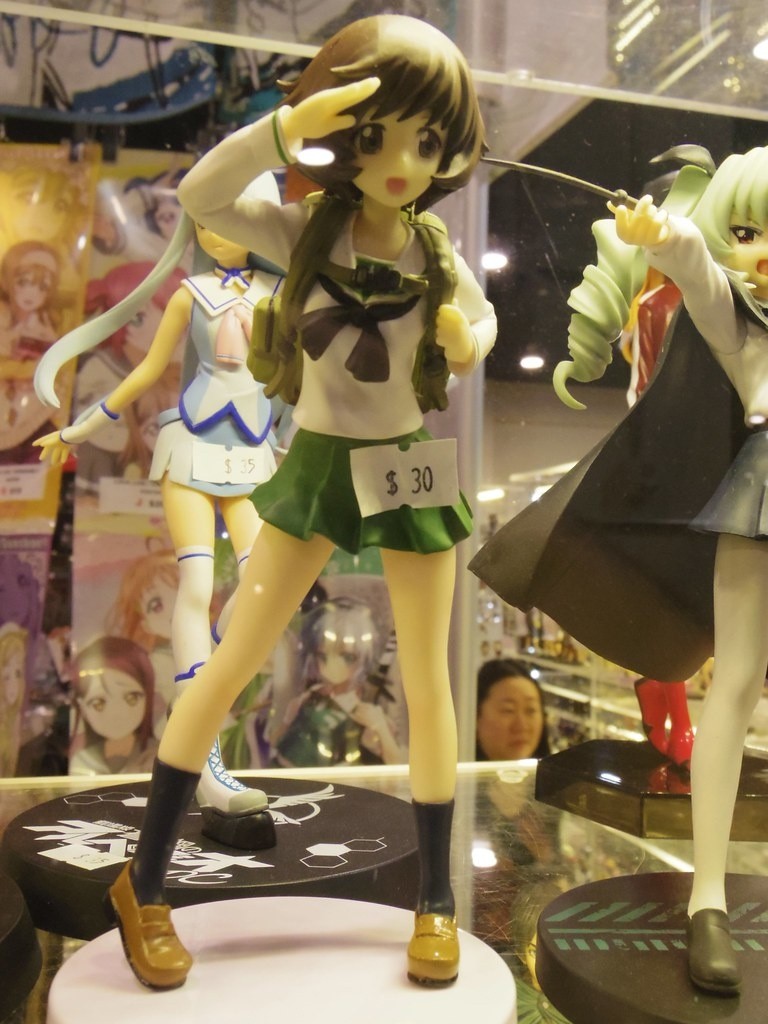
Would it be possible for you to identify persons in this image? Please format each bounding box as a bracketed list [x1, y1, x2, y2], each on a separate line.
[32, 171, 292, 820]
[108, 16, 499, 991]
[475, 658, 552, 761]
[554, 144, 768, 992]
